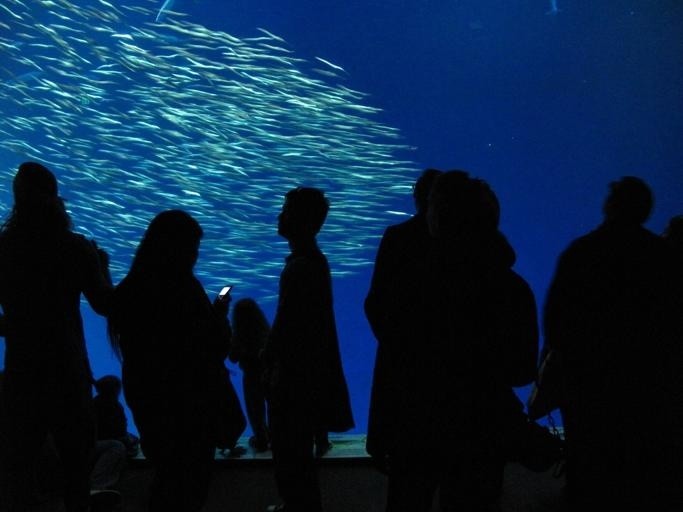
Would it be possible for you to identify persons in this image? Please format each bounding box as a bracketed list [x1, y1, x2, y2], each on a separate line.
[527, 177, 683, 512]
[365, 170, 539, 512]
[229, 298, 271, 446]
[108, 212, 247, 512]
[0, 164, 114, 512]
[370, 169, 441, 286]
[92, 376, 139, 456]
[658, 216, 683, 247]
[315, 432, 331, 457]
[256, 189, 355, 512]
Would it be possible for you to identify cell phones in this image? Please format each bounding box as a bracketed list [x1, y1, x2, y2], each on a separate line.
[217, 285, 233, 301]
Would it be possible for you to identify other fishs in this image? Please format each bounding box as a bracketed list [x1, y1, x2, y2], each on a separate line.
[0, 0, 425, 306]
